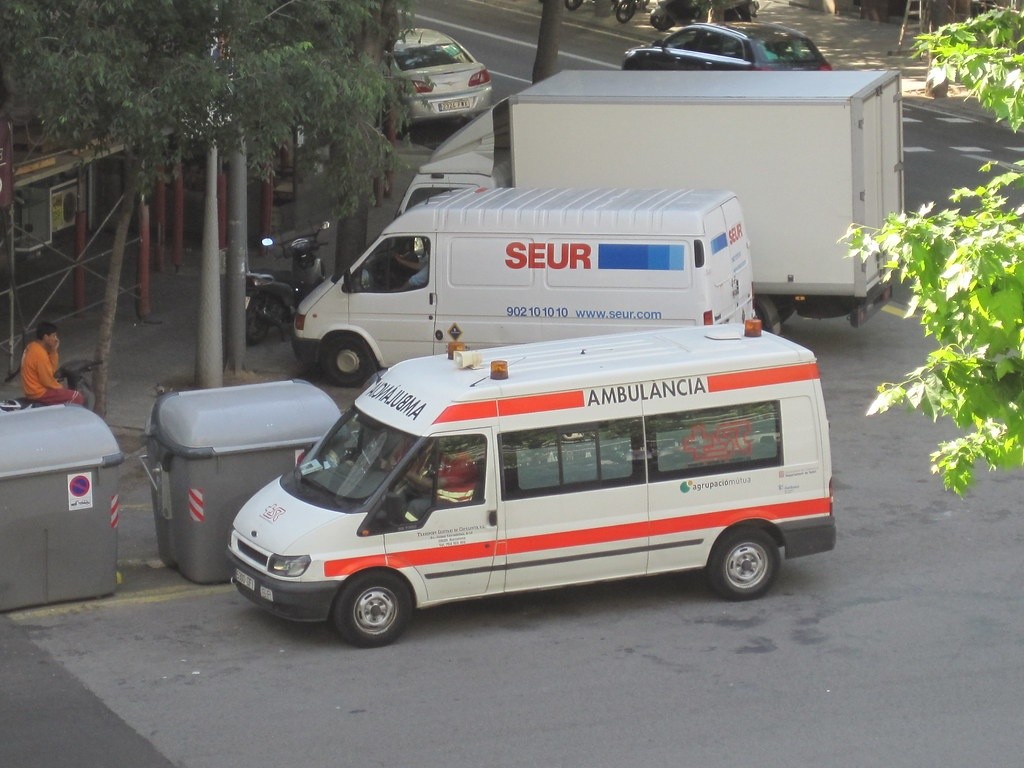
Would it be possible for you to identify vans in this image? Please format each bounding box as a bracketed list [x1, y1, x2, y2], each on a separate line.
[226, 320, 838, 648]
[292, 187, 756, 388]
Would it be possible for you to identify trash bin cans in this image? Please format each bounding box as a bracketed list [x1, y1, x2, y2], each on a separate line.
[147, 378, 351, 585]
[1, 403, 124, 612]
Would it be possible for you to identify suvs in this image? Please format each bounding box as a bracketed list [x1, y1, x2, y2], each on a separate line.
[621, 21, 832, 70]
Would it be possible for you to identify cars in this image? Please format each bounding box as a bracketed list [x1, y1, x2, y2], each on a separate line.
[384, 28, 493, 131]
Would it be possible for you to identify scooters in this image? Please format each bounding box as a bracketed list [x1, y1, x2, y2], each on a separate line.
[246, 220, 333, 345]
[5, 356, 105, 425]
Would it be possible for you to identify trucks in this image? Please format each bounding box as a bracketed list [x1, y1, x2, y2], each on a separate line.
[396, 67, 904, 332]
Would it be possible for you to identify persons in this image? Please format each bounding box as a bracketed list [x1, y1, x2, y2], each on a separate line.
[387, 238, 431, 293]
[21, 321, 85, 408]
[394, 437, 481, 531]
